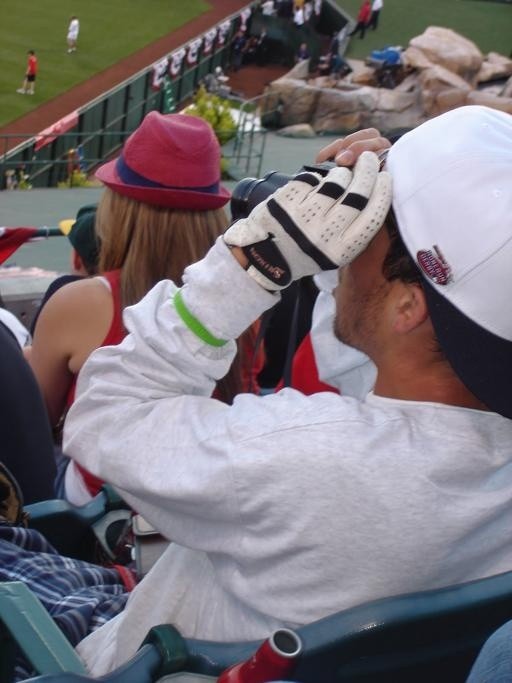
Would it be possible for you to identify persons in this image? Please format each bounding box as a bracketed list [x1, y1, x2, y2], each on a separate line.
[1, 2, 512, 683]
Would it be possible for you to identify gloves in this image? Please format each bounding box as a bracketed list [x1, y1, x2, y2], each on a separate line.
[221, 150, 394, 293]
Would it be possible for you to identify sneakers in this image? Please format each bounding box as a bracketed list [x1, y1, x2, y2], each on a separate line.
[17, 88, 25, 94]
[27, 89, 35, 95]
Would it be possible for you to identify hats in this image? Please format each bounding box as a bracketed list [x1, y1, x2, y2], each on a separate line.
[59, 203, 101, 274]
[95, 109, 233, 211]
[383, 102, 511, 418]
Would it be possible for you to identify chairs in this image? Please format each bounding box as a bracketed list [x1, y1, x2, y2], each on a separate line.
[1, 568, 512, 683]
[22, 482, 130, 562]
